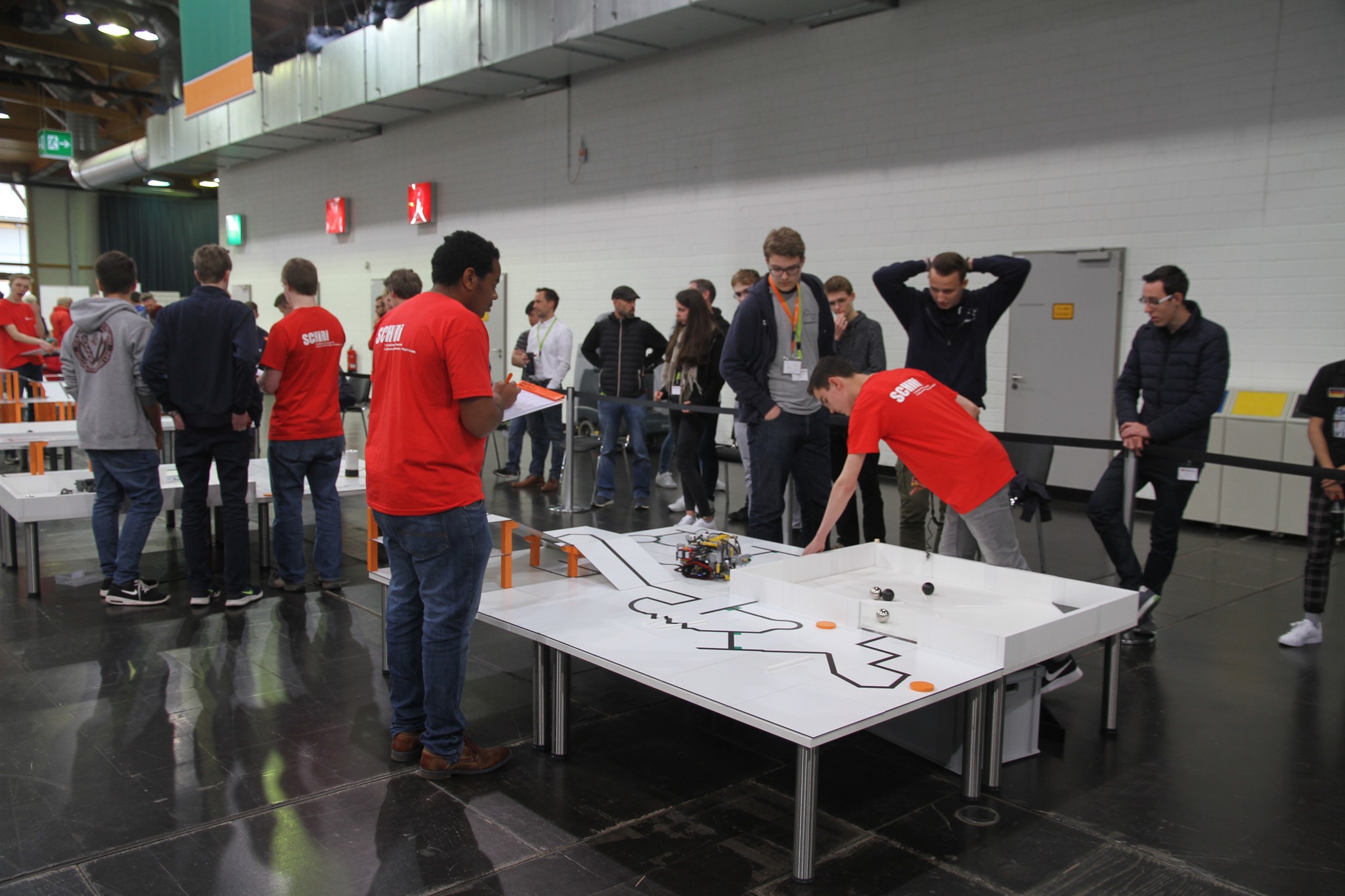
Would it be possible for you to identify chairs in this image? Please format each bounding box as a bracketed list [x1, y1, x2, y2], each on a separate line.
[573, 405, 635, 503]
[715, 421, 742, 526]
[987, 443, 1054, 574]
[341, 374, 368, 443]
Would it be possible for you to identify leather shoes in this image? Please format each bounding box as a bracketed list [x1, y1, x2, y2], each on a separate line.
[390, 730, 422, 763]
[420, 741, 512, 776]
[512, 474, 544, 488]
[542, 477, 558, 492]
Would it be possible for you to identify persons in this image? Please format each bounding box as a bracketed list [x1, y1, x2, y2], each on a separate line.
[1086, 263, 1231, 639]
[0, 273, 63, 465]
[139, 243, 263, 607]
[50, 296, 73, 345]
[22, 293, 50, 338]
[132, 291, 163, 326]
[511, 287, 572, 492]
[245, 301, 268, 377]
[718, 226, 834, 552]
[255, 258, 346, 591]
[493, 301, 540, 479]
[729, 268, 760, 522]
[375, 267, 422, 329]
[872, 251, 1031, 550]
[274, 290, 293, 318]
[58, 251, 170, 605]
[1278, 359, 1345, 647]
[824, 276, 887, 550]
[653, 289, 725, 529]
[582, 286, 667, 510]
[802, 353, 1083, 696]
[365, 230, 522, 775]
[656, 279, 730, 512]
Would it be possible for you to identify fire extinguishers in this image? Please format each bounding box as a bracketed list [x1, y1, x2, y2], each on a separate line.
[348, 344, 357, 371]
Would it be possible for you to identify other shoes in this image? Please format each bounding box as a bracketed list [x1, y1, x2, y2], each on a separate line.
[262, 565, 305, 592]
[728, 494, 750, 522]
[3, 453, 20, 465]
[1134, 616, 1158, 635]
[313, 572, 342, 589]
[1138, 586, 1163, 618]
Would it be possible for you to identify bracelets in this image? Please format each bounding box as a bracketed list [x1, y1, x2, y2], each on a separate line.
[965, 256, 971, 273]
[927, 257, 932, 271]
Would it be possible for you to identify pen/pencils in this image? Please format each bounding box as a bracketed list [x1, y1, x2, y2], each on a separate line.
[50, 341, 56, 355]
[505, 373, 512, 383]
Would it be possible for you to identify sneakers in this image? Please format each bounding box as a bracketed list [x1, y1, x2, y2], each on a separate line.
[494, 467, 518, 478]
[105, 580, 171, 605]
[692, 517, 717, 529]
[595, 495, 614, 507]
[667, 496, 685, 512]
[100, 579, 158, 597]
[1040, 655, 1083, 696]
[634, 497, 649, 510]
[225, 584, 264, 607]
[1278, 618, 1322, 646]
[674, 513, 697, 526]
[190, 585, 221, 605]
[715, 480, 725, 491]
[656, 472, 678, 489]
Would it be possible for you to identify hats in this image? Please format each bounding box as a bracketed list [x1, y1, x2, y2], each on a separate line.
[610, 286, 640, 300]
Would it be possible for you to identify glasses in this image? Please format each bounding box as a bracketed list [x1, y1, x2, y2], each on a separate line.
[734, 289, 746, 301]
[1139, 295, 1172, 306]
[768, 261, 802, 278]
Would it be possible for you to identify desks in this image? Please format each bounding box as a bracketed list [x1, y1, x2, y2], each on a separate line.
[368, 523, 1141, 882]
[0, 379, 368, 599]
